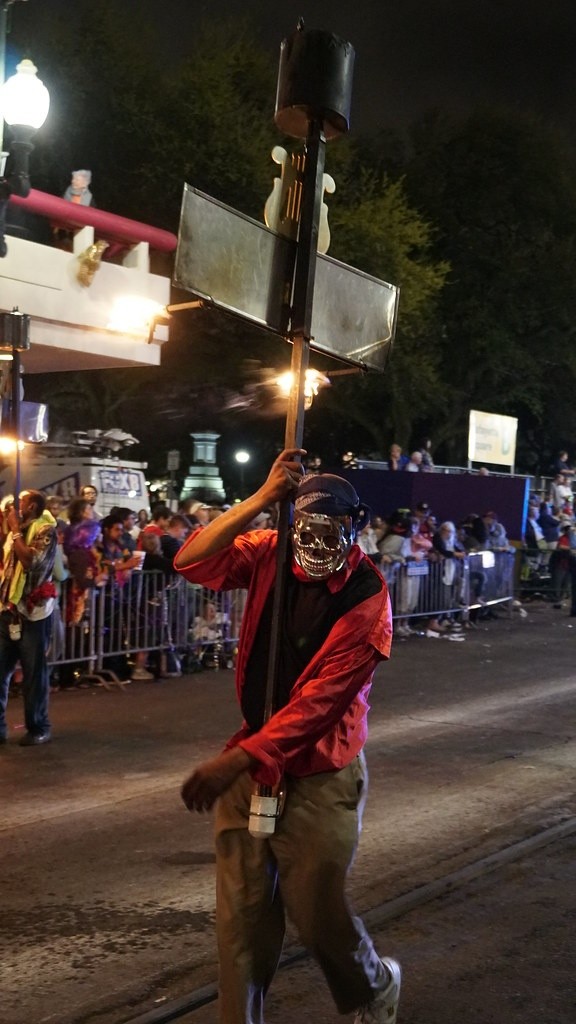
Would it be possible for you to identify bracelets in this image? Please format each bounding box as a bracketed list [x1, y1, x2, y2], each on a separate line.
[12, 532, 23, 539]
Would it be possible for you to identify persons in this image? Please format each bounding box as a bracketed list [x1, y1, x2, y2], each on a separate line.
[173, 449, 401, 1023]
[0, 486, 509, 748]
[524, 452, 576, 608]
[388, 438, 434, 473]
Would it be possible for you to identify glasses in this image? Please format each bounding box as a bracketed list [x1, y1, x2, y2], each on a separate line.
[84, 491, 97, 496]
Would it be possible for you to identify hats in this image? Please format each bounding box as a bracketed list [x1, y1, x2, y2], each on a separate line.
[558, 520, 572, 530]
[189, 501, 212, 515]
[559, 536, 570, 546]
[417, 501, 431, 512]
[482, 510, 498, 519]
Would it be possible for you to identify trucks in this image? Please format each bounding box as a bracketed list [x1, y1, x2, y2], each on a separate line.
[0, 429, 151, 518]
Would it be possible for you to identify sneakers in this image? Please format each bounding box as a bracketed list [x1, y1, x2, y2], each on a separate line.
[130, 667, 154, 680]
[353, 955, 403, 1024]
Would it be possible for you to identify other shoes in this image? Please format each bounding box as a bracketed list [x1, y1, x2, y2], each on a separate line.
[474, 595, 486, 604]
[395, 624, 416, 636]
[441, 617, 461, 628]
[553, 604, 561, 609]
[425, 620, 450, 632]
[80, 668, 131, 686]
[479, 608, 498, 621]
[458, 598, 469, 609]
[58, 677, 90, 691]
[18, 726, 51, 746]
